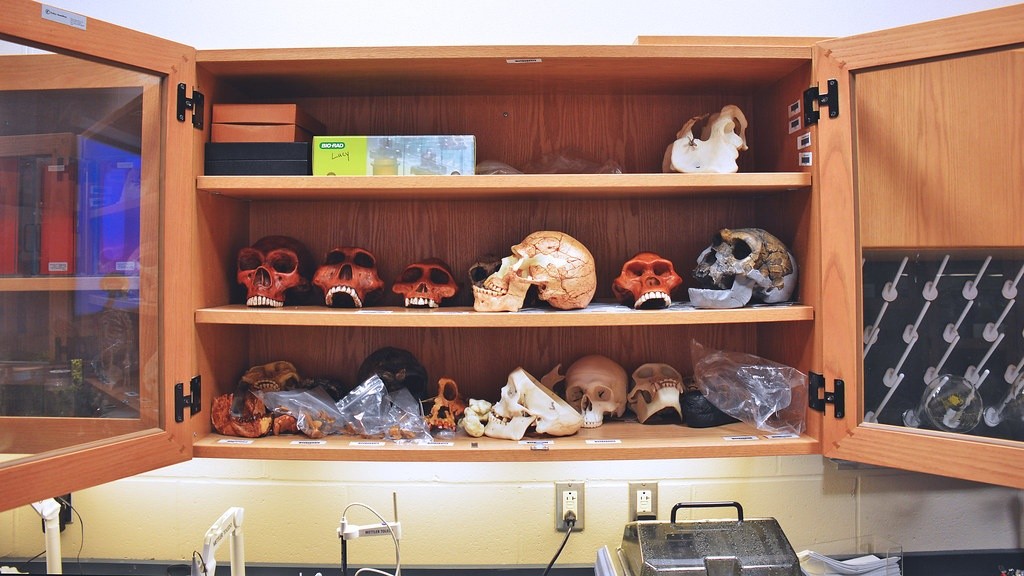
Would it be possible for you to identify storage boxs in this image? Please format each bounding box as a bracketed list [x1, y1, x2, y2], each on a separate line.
[211, 104, 327, 142]
[204, 142, 312, 175]
[312, 135, 475, 177]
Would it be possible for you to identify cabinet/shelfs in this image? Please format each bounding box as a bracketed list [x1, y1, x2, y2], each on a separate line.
[0, 1, 1024, 495]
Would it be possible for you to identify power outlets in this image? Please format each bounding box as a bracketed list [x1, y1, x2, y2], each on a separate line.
[54, 493, 74, 524]
[629, 481, 658, 521]
[555, 482, 584, 532]
